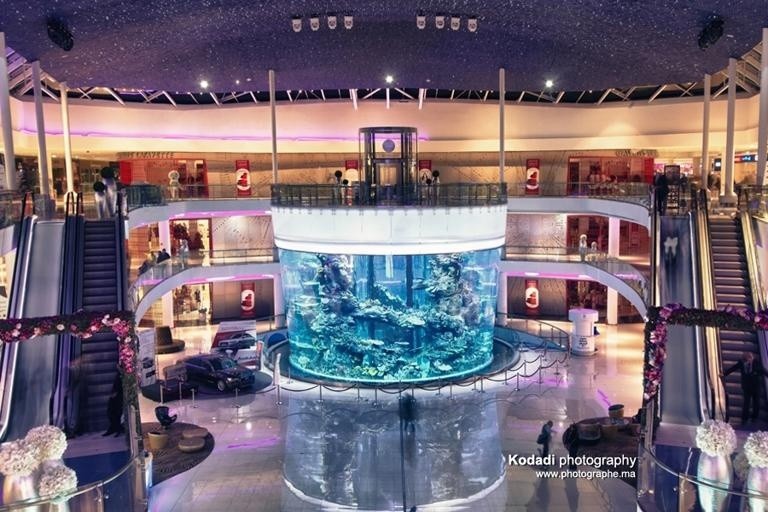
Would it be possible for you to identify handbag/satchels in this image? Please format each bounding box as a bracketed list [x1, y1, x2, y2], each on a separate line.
[536, 434, 546, 444]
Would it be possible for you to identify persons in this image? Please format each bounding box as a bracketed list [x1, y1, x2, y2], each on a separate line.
[566, 468, 579, 512]
[139, 248, 172, 276]
[399, 393, 416, 433]
[566, 424, 579, 471]
[542, 420, 554, 466]
[580, 234, 587, 262]
[537, 471, 550, 510]
[652, 169, 686, 215]
[591, 241, 598, 263]
[103, 364, 124, 438]
[718, 351, 766, 426]
[65, 353, 84, 434]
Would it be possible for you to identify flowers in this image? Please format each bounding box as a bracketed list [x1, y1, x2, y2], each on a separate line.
[0, 424, 78, 504]
[695, 418, 768, 482]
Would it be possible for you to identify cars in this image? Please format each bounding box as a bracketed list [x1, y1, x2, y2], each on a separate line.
[176, 353, 255, 395]
[217, 332, 255, 350]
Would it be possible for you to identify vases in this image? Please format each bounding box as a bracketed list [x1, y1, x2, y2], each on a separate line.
[2, 460, 70, 512]
[697, 451, 768, 512]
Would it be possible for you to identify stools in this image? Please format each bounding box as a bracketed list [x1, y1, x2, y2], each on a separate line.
[178, 427, 208, 453]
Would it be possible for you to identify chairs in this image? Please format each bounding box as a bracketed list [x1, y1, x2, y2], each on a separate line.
[147, 407, 177, 450]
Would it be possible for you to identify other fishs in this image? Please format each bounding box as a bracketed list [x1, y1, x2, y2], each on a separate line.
[408, 318, 425, 325]
[369, 340, 384, 345]
[394, 342, 409, 345]
